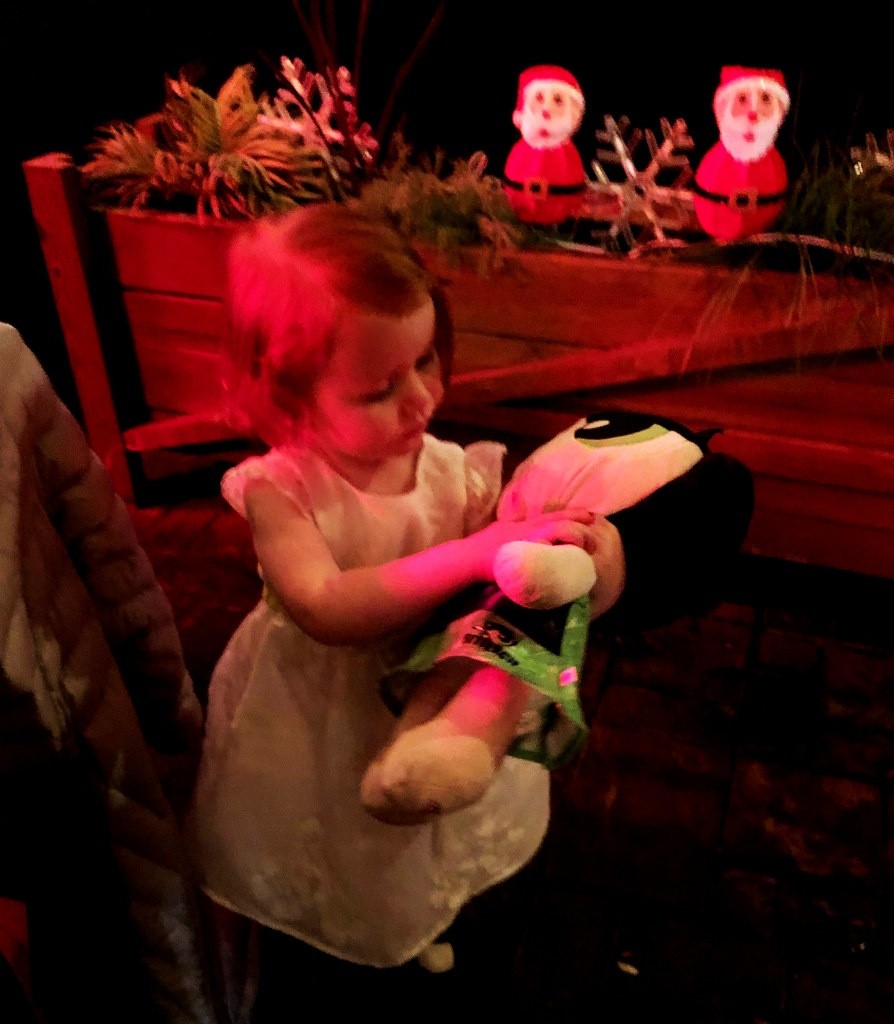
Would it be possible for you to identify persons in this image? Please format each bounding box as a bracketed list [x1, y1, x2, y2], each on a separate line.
[0, 203, 626, 1023]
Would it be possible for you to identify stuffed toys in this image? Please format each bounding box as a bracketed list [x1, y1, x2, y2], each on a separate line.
[360, 410, 755, 828]
[503, 64, 792, 245]
